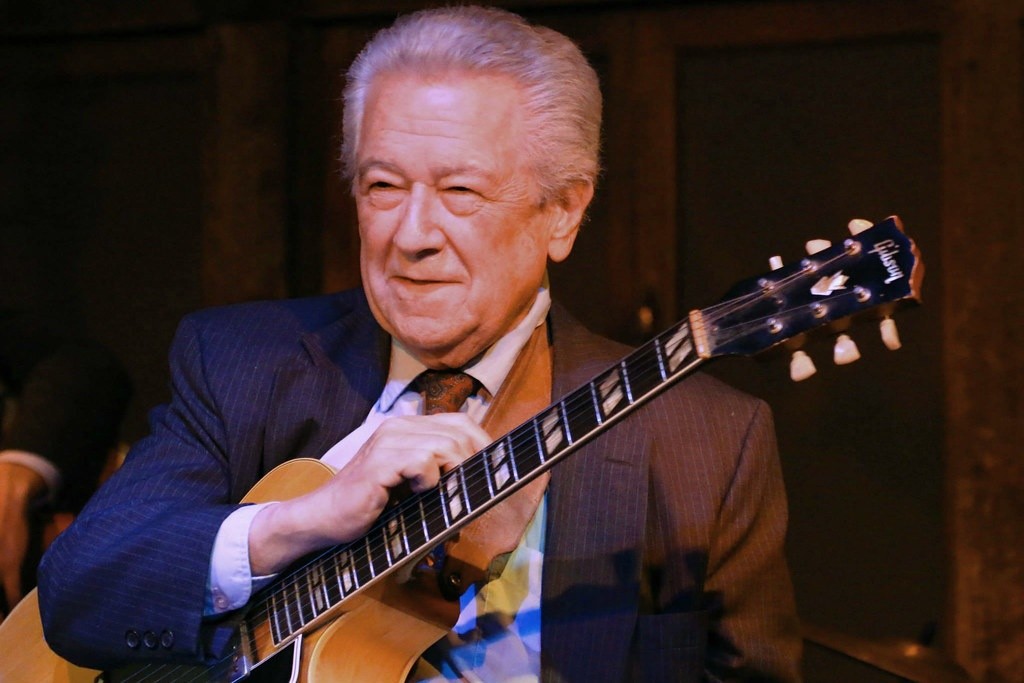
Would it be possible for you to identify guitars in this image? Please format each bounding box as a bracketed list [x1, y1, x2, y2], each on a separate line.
[0, 213, 924, 680]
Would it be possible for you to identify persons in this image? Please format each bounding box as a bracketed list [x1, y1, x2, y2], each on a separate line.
[36, 7, 806, 683]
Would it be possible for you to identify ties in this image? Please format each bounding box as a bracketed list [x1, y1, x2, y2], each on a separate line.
[416, 367, 474, 416]
